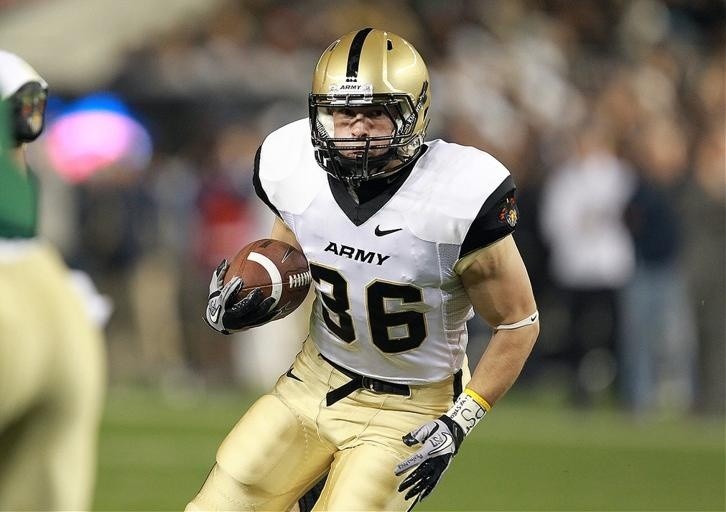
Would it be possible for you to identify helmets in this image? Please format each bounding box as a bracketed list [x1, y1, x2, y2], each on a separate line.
[312, 27, 430, 180]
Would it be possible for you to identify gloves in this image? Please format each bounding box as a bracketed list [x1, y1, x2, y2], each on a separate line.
[395, 414, 463, 501]
[202, 259, 279, 334]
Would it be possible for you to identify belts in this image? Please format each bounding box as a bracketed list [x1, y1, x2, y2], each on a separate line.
[319, 353, 410, 407]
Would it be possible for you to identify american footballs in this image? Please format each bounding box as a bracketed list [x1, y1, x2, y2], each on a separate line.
[222, 239, 311, 322]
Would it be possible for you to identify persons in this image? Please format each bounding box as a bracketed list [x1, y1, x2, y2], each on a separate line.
[181, 28, 541, 508]
[1, 234, 106, 511]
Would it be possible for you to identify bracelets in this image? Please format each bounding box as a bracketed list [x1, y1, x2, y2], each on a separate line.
[447, 387, 491, 437]
